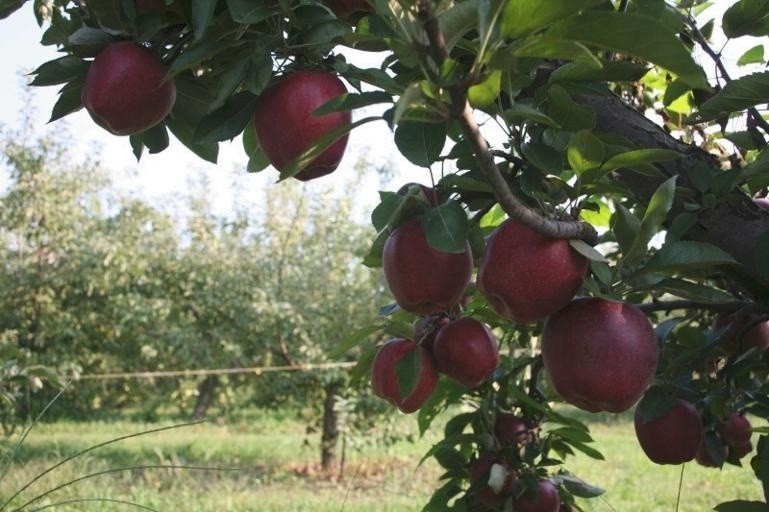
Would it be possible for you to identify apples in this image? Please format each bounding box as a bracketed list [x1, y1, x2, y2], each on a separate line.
[82, 40, 174, 135]
[651, 304, 769, 377]
[635, 396, 706, 464]
[373, 182, 500, 412]
[542, 297, 661, 412]
[253, 69, 350, 180]
[745, 189, 769, 221]
[699, 413, 753, 468]
[468, 412, 565, 508]
[474, 205, 590, 324]
